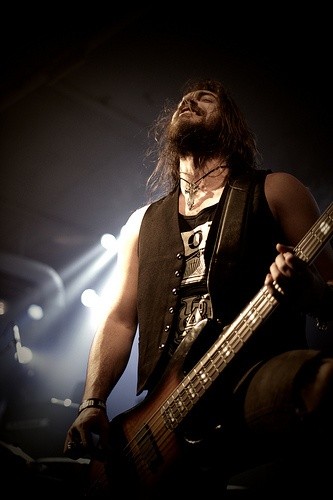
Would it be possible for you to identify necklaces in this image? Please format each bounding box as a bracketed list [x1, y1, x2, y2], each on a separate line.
[179, 165, 230, 210]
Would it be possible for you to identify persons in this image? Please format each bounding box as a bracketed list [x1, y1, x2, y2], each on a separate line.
[64, 79, 333, 500]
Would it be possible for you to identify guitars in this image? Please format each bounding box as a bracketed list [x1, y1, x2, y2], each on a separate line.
[87, 201, 333, 500]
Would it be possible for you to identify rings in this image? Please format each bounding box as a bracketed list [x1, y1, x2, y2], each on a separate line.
[68, 442, 77, 449]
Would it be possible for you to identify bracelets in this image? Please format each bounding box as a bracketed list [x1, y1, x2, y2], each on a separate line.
[78, 398, 107, 415]
[316, 323, 333, 337]
